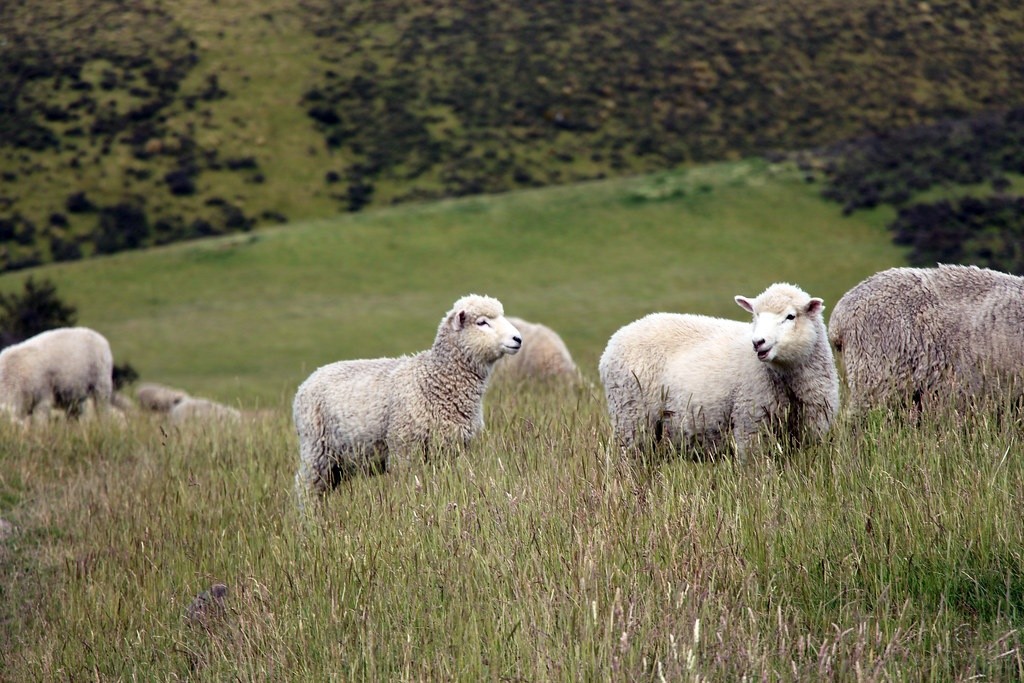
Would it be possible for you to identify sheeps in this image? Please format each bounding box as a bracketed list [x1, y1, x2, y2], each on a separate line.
[0, 328, 241, 427]
[596, 281, 841, 492]
[490, 317, 598, 403]
[827, 263, 1024, 429]
[291, 293, 522, 525]
